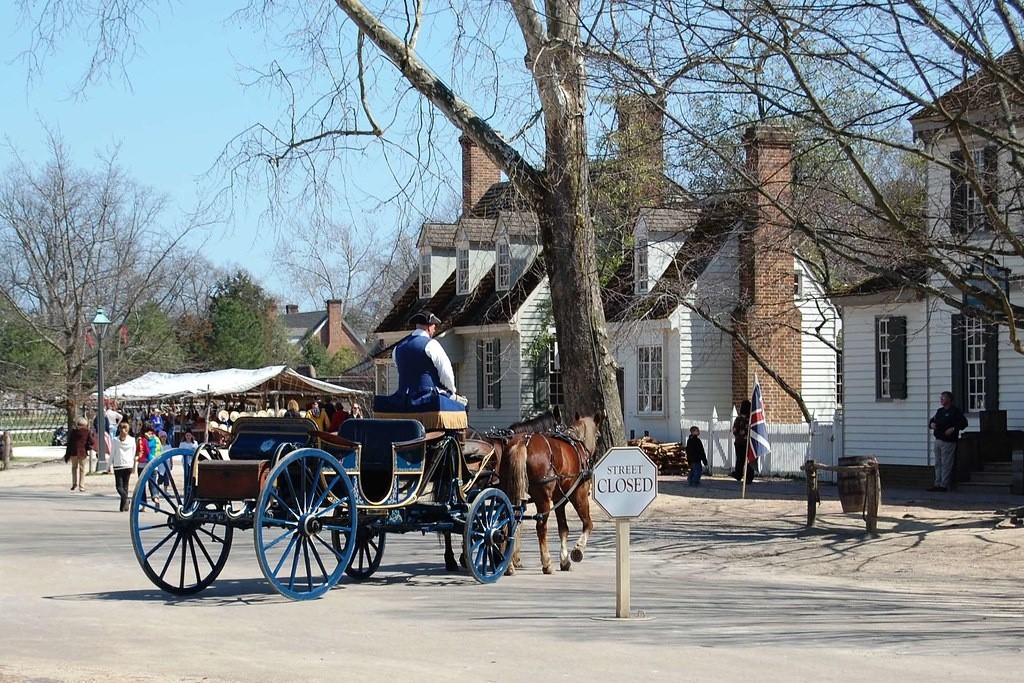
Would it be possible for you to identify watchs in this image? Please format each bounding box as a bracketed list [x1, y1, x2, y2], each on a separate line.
[952, 427, 955, 431]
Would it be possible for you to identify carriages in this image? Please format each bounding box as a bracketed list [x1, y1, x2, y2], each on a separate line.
[92, 363, 609, 602]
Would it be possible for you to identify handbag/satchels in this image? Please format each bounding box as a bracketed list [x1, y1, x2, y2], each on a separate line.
[86, 432, 93, 450]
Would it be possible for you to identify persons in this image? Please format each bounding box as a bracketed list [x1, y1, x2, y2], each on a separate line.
[54, 427, 64, 445]
[92, 404, 200, 513]
[927, 391, 968, 492]
[66, 417, 95, 491]
[284, 395, 363, 432]
[731, 400, 754, 484]
[686, 426, 708, 487]
[392, 310, 462, 398]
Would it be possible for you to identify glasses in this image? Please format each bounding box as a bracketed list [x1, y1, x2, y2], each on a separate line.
[353, 407, 359, 409]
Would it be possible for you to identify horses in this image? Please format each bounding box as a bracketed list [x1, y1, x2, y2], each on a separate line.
[430, 404, 568, 573]
[491, 412, 601, 576]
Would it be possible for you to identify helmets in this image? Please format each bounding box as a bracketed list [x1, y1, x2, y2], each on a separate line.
[407, 311, 441, 325]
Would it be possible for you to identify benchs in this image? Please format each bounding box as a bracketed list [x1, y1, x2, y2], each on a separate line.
[227, 417, 362, 475]
[372, 394, 469, 432]
[339, 417, 428, 471]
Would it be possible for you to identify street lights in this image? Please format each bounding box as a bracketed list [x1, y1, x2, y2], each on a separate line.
[90, 308, 113, 473]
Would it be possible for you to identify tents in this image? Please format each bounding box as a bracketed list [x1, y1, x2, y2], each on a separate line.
[90, 365, 373, 442]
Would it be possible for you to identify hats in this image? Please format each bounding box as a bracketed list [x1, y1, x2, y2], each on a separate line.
[159, 430, 167, 438]
[154, 408, 162, 413]
[312, 400, 318, 405]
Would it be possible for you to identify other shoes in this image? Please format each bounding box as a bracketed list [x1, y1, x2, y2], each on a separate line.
[693, 482, 698, 487]
[155, 502, 160, 512]
[164, 491, 170, 497]
[120, 506, 123, 512]
[746, 480, 752, 484]
[139, 508, 146, 512]
[71, 484, 77, 490]
[736, 475, 742, 480]
[687, 480, 692, 485]
[928, 486, 947, 491]
[124, 499, 129, 511]
[80, 486, 85, 491]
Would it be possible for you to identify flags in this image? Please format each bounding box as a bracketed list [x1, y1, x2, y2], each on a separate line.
[745, 372, 772, 465]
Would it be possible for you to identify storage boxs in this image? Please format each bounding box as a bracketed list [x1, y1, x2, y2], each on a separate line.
[197, 457, 278, 499]
